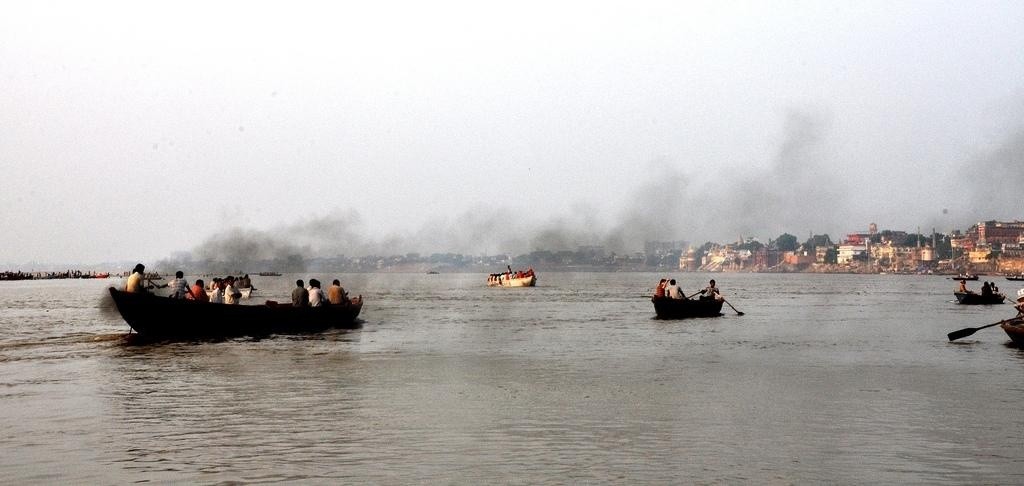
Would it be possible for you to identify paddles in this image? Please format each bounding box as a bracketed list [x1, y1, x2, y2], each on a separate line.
[714, 292, 744, 315]
[947, 316, 1024, 341]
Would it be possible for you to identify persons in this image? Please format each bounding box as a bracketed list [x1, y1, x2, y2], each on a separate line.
[305, 277, 317, 292]
[990, 281, 999, 294]
[486, 263, 536, 286]
[124, 263, 258, 304]
[699, 278, 719, 300]
[0, 266, 283, 281]
[291, 279, 310, 305]
[982, 280, 992, 295]
[1014, 296, 1024, 327]
[1004, 269, 1023, 281]
[955, 269, 978, 279]
[655, 277, 670, 297]
[959, 279, 972, 293]
[308, 280, 331, 307]
[327, 277, 348, 304]
[665, 278, 688, 299]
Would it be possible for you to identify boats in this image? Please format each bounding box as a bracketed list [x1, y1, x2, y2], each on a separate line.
[951, 274, 1024, 348]
[108, 271, 364, 341]
[0, 271, 109, 280]
[652, 294, 725, 319]
[486, 275, 537, 289]
